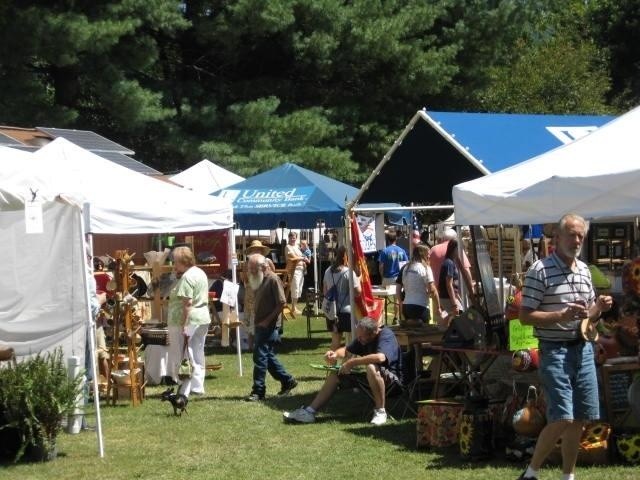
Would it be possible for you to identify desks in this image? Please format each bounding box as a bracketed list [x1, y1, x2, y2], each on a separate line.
[391, 325, 447, 400]
[432, 345, 513, 398]
[372, 285, 403, 326]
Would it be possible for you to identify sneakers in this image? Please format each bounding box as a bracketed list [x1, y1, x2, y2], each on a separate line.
[370, 408, 388, 425]
[276, 379, 298, 398]
[245, 392, 265, 401]
[283, 406, 315, 424]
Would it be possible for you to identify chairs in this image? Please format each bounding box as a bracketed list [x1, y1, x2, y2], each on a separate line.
[348, 347, 416, 419]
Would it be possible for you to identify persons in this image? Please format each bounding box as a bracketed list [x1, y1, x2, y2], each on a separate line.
[440, 241, 465, 316]
[322, 246, 362, 347]
[299, 240, 311, 264]
[402, 244, 444, 328]
[427, 229, 476, 315]
[282, 318, 406, 425]
[284, 232, 310, 315]
[246, 254, 298, 402]
[517, 213, 614, 480]
[244, 239, 271, 255]
[379, 230, 409, 287]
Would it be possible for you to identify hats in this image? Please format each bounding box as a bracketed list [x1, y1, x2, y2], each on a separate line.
[241, 239, 270, 256]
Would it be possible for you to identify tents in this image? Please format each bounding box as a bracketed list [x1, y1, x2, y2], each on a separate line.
[345, 108, 640, 394]
[202, 164, 370, 320]
[452, 100, 638, 450]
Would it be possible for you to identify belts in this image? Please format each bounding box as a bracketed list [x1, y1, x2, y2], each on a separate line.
[537, 336, 589, 348]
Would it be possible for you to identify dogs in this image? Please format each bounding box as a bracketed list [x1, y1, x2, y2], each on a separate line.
[160, 384, 188, 417]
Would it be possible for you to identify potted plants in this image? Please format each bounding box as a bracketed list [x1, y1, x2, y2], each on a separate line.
[0, 346, 97, 464]
